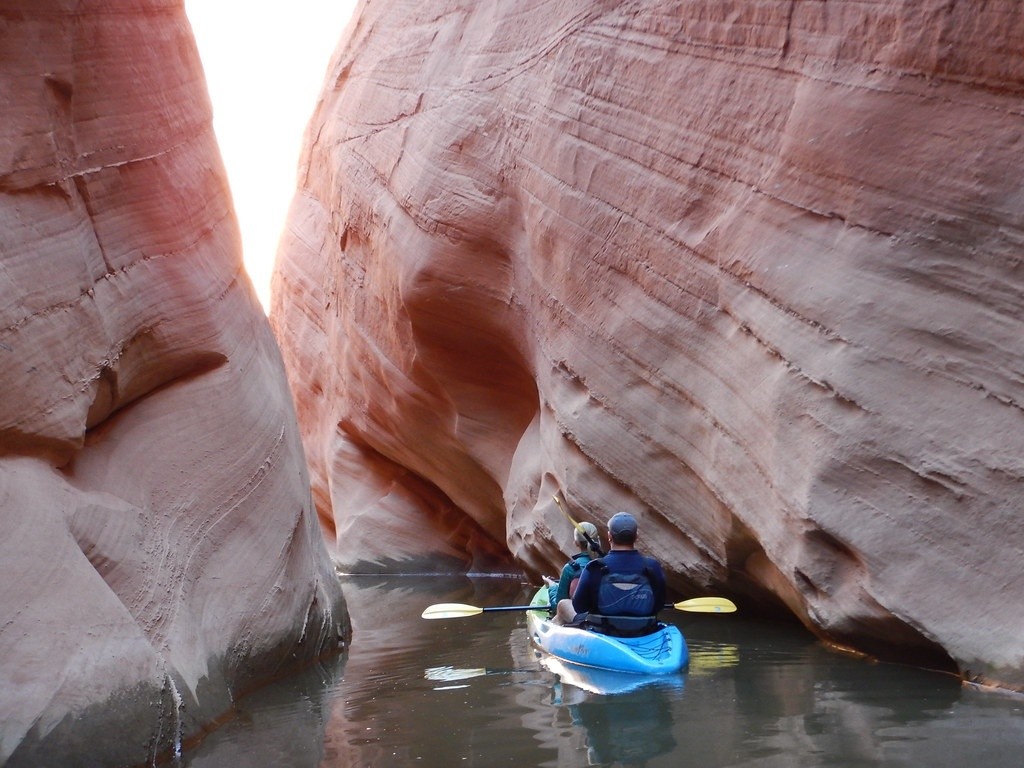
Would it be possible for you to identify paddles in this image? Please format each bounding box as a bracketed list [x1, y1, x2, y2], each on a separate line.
[554, 492, 605, 557]
[420, 596, 736, 621]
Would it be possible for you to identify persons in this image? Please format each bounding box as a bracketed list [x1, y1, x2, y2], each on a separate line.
[541, 521, 601, 611]
[557, 511, 668, 625]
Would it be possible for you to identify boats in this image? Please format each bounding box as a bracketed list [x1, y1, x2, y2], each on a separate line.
[531, 647, 685, 698]
[527, 583, 692, 678]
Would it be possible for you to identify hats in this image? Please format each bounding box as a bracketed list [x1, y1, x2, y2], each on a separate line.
[606, 512, 638, 535]
